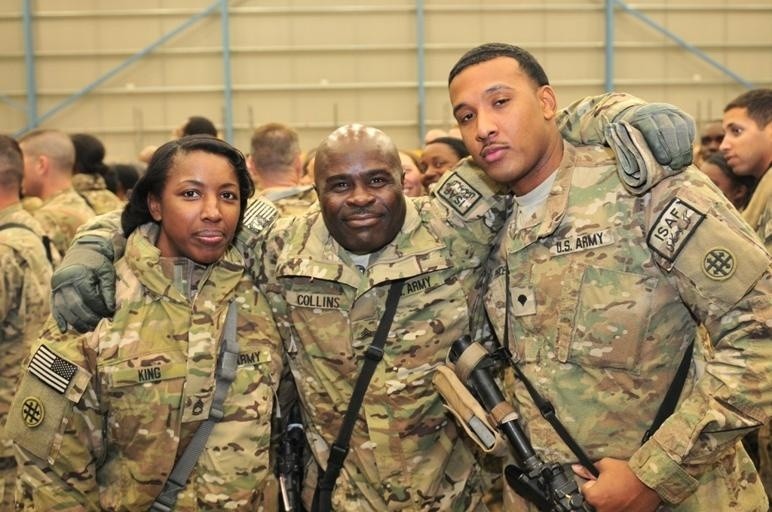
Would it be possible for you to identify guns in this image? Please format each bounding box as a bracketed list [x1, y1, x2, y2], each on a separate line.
[276, 401, 306, 511]
[448, 334, 596, 512]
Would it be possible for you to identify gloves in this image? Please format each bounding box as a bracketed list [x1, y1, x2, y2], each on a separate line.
[625, 99, 699, 171]
[51, 234, 117, 333]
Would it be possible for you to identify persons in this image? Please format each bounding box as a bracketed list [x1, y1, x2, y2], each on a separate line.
[0, 42, 772, 511]
